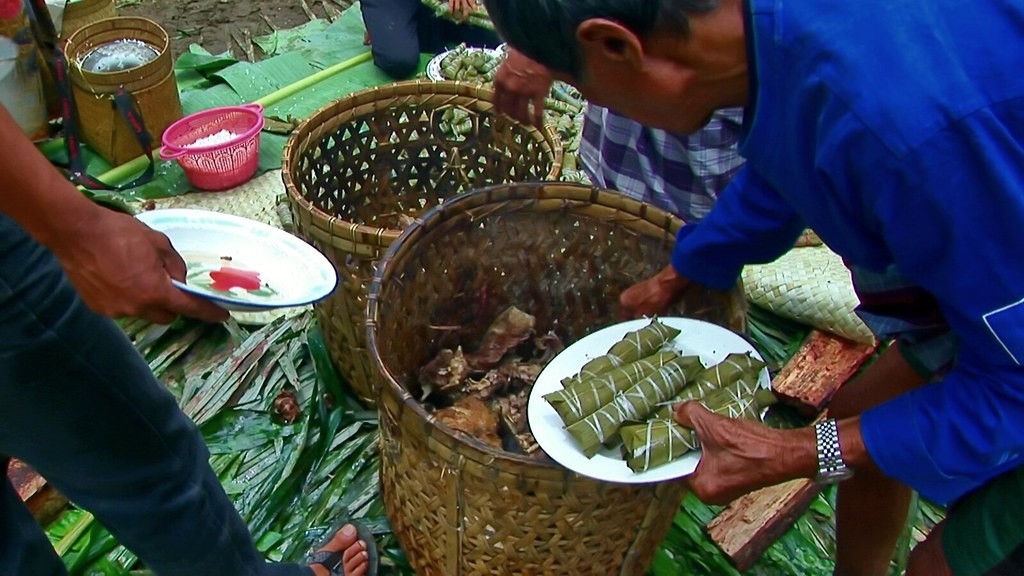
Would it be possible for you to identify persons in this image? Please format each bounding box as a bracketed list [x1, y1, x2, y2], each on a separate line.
[482, 0, 1024, 576]
[360, 0, 500, 79]
[0, 105, 380, 576]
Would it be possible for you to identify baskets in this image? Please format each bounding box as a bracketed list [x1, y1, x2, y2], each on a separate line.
[159, 102, 264, 191]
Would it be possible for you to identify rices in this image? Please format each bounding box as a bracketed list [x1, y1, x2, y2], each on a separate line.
[176, 129, 250, 172]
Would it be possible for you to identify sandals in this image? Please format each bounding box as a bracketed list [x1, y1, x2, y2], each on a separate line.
[304, 520, 379, 576]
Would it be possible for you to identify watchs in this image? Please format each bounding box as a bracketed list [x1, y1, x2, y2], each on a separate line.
[814, 418, 855, 486]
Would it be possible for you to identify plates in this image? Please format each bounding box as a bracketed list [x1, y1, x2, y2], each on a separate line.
[132, 208, 338, 312]
[425, 41, 508, 82]
[527, 317, 772, 483]
